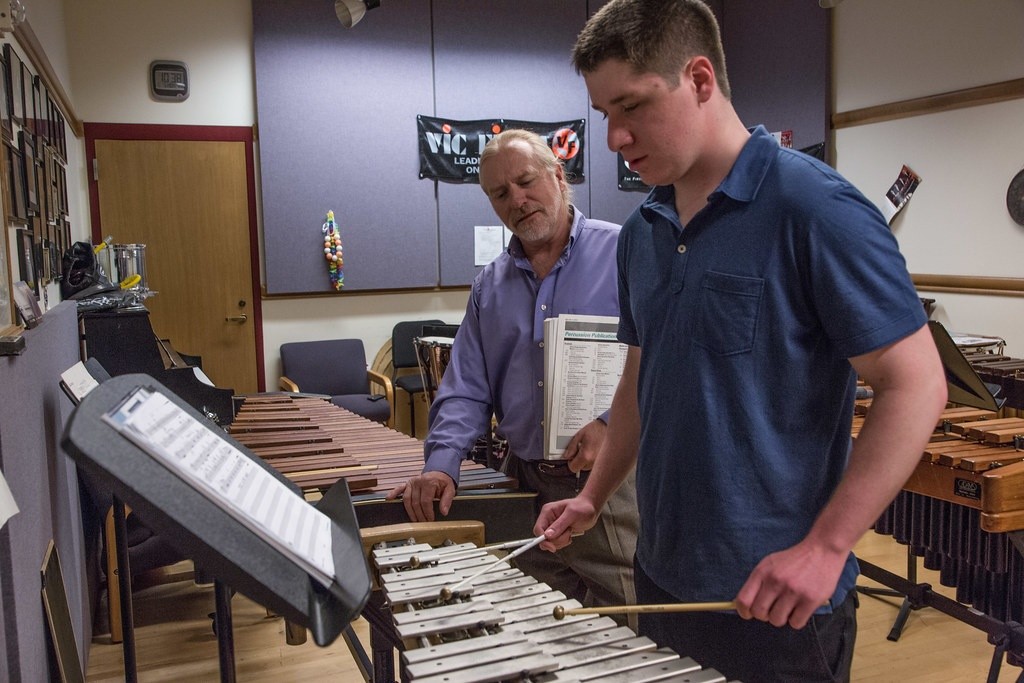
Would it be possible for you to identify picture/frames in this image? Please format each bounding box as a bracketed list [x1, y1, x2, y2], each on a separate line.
[0, 42, 72, 301]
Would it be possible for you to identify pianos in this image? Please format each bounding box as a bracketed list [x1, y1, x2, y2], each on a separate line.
[83, 302, 235, 428]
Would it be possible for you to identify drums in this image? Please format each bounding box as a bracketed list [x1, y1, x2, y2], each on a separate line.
[413, 336, 455, 391]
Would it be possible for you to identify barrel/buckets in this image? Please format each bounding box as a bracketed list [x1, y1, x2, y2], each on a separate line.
[91, 243, 146, 302]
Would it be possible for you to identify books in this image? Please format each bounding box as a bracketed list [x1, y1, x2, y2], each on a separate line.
[541, 314, 629, 461]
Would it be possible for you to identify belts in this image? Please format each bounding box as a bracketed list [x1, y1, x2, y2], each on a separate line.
[534, 461, 574, 477]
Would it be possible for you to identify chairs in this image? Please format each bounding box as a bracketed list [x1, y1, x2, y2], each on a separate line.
[392, 320, 448, 437]
[279, 338, 395, 429]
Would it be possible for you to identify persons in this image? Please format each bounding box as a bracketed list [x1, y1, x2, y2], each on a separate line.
[532, 0, 948, 683]
[385, 128, 637, 631]
